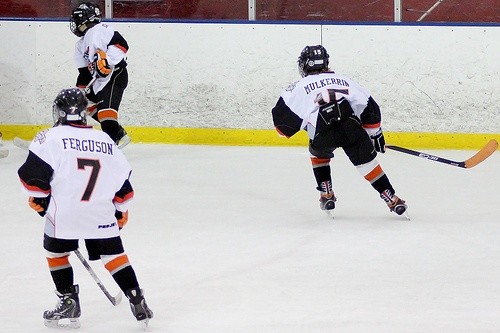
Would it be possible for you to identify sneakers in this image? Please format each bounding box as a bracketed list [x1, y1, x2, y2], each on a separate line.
[107, 125, 131, 150]
[316, 180, 337, 219]
[380, 189, 411, 222]
[43, 284, 82, 329]
[124, 288, 153, 332]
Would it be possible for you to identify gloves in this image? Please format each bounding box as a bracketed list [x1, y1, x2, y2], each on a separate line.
[370, 127, 386, 153]
[88, 50, 111, 78]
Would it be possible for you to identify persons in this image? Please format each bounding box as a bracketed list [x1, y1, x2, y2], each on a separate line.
[70, 2, 133, 150]
[271, 44, 411, 220]
[17, 88, 153, 332]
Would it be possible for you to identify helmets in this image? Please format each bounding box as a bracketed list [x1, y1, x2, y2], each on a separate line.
[70, 2, 101, 37]
[298, 45, 330, 78]
[52, 88, 88, 126]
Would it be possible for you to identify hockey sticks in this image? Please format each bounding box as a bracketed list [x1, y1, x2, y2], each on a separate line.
[13, 77, 98, 151]
[44, 212, 123, 307]
[384, 138, 499, 169]
